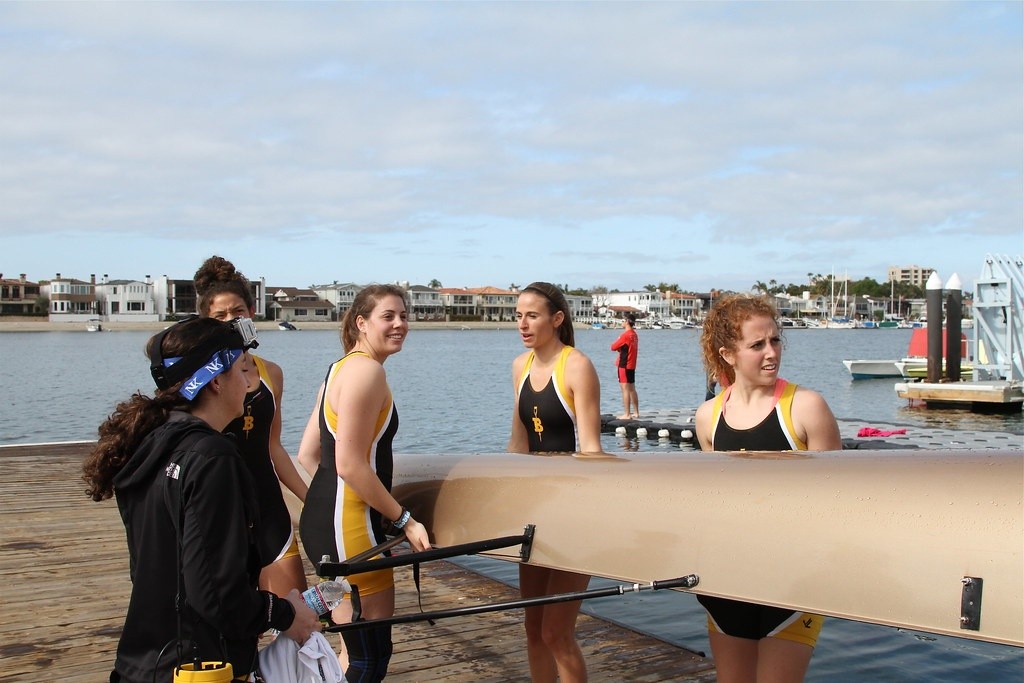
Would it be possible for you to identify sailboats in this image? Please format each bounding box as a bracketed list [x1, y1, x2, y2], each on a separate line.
[827, 268, 857, 329]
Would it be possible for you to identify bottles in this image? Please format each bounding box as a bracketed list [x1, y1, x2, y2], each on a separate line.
[270, 579, 351, 637]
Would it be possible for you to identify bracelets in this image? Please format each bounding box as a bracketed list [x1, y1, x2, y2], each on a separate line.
[392, 508, 410, 529]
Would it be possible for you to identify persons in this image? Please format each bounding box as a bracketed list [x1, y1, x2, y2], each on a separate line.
[507, 282, 601, 683]
[82, 314, 326, 683]
[694, 294, 842, 683]
[611, 314, 640, 419]
[192, 255, 308, 598]
[298, 283, 432, 683]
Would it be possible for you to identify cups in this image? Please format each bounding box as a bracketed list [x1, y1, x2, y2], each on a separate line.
[172, 658, 234, 682]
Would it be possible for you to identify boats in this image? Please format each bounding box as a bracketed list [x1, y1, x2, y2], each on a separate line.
[668, 315, 695, 330]
[842, 359, 903, 380]
[907, 364, 972, 380]
[777, 316, 827, 330]
[267, 447, 1023, 651]
[592, 324, 606, 329]
[856, 306, 973, 331]
[649, 320, 670, 329]
[278, 321, 296, 331]
[894, 329, 968, 381]
[86, 323, 103, 331]
